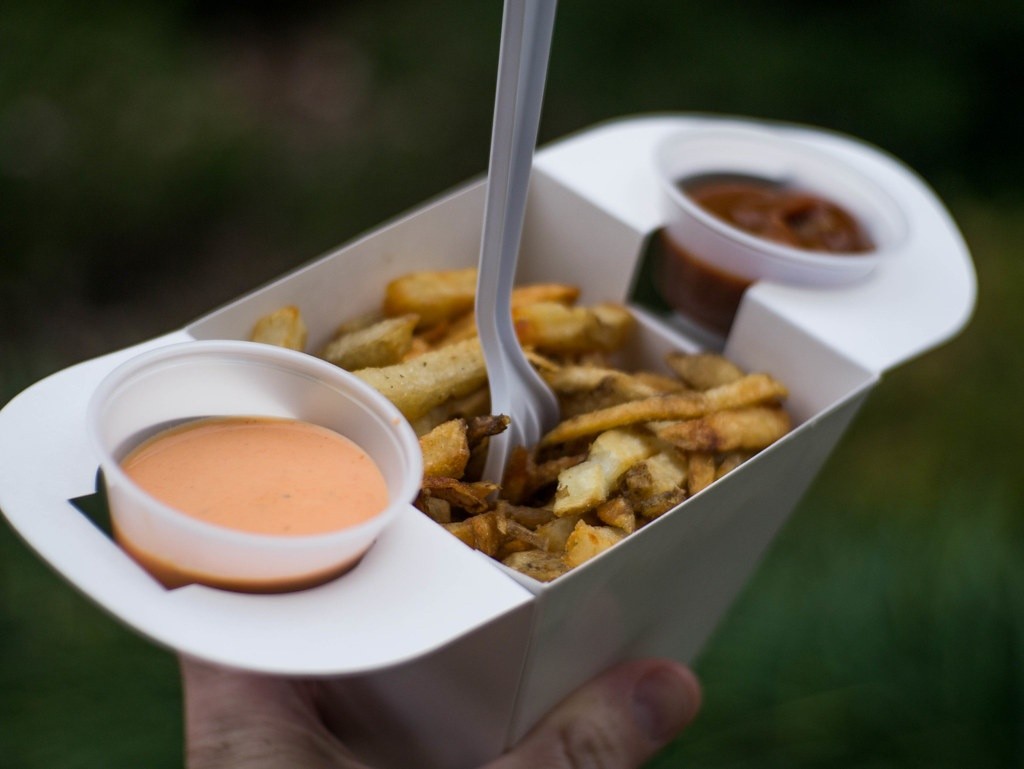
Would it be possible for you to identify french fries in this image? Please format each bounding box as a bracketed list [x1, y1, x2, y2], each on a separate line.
[245, 267, 795, 585]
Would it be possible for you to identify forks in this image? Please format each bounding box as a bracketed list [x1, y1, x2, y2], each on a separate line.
[474, 0, 562, 504]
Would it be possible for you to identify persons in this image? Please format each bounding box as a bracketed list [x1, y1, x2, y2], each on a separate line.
[182, 651, 702, 769]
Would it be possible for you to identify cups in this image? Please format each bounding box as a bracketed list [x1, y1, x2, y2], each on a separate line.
[83, 338, 426, 594]
[657, 127, 917, 338]
